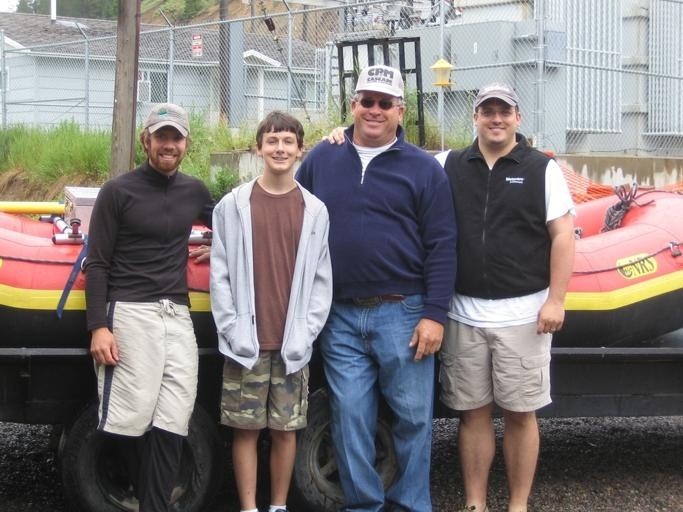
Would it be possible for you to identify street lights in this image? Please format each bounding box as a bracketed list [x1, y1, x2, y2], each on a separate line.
[430, 59, 457, 152]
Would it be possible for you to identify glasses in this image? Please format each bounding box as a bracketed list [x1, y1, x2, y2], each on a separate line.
[360, 97, 392, 109]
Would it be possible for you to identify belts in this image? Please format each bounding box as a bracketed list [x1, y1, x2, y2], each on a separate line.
[352, 294, 404, 306]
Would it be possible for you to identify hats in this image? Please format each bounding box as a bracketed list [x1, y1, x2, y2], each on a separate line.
[143, 103, 189, 137]
[473, 82, 519, 113]
[355, 64, 404, 99]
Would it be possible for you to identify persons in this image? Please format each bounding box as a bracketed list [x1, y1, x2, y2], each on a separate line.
[82, 102, 216, 510]
[208, 107, 335, 512]
[318, 83, 572, 511]
[185, 65, 458, 511]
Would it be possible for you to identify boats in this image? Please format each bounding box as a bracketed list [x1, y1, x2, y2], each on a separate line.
[0, 187, 682, 347]
